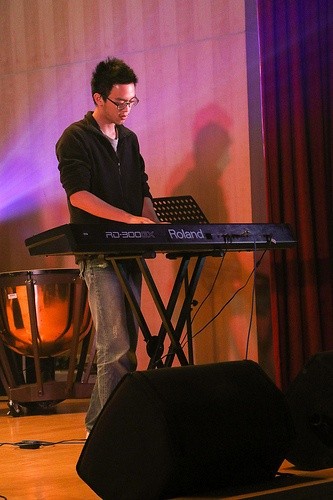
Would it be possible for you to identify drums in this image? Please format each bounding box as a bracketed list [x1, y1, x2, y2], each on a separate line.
[0, 267, 95, 411]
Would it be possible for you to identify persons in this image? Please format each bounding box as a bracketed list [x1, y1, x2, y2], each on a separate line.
[55, 55, 172, 440]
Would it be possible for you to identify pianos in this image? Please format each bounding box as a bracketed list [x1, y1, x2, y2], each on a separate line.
[25, 220, 299, 255]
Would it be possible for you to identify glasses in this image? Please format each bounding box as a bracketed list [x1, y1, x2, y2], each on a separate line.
[106, 96, 139, 110]
